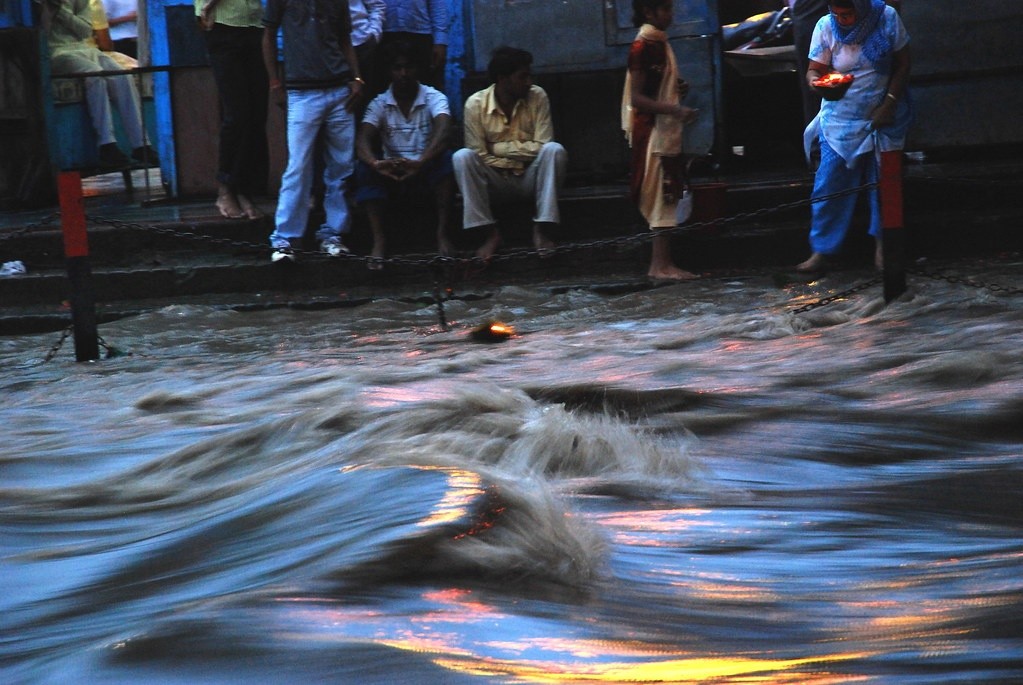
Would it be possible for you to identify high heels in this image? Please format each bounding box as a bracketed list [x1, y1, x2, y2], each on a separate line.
[215, 198, 245, 218]
[239, 206, 266, 220]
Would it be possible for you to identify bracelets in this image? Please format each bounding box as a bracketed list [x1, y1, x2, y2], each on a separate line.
[676, 103, 680, 116]
[269, 78, 280, 86]
[887, 92, 896, 101]
[355, 77, 364, 85]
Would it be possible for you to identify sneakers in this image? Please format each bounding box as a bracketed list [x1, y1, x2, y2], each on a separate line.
[270, 248, 295, 265]
[319, 236, 351, 257]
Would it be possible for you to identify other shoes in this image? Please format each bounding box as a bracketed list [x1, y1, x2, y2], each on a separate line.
[97, 148, 138, 170]
[131, 146, 160, 166]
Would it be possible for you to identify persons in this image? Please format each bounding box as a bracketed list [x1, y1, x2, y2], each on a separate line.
[260, 0, 364, 265]
[194, 0, 270, 220]
[450, 47, 569, 264]
[620, 0, 710, 279]
[794, 0, 911, 274]
[348, 0, 471, 124]
[356, 41, 458, 272]
[41, 0, 161, 167]
